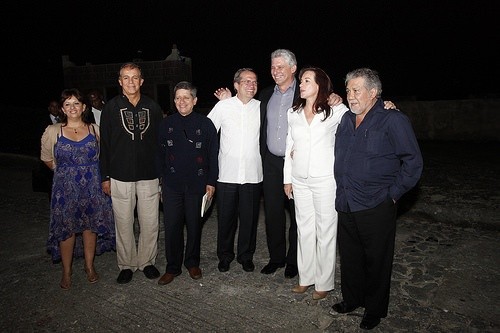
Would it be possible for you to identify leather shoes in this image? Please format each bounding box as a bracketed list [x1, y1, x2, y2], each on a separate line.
[291, 285, 307, 293]
[158, 270, 182, 285]
[312, 290, 328, 299]
[189, 266, 202, 280]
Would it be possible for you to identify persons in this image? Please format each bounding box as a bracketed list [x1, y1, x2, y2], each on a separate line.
[283, 67, 401, 300]
[330, 68, 424, 330]
[41, 88, 116, 290]
[48, 100, 66, 125]
[87, 90, 105, 126]
[213, 49, 343, 277]
[158, 81, 218, 285]
[204, 67, 265, 272]
[99, 62, 163, 283]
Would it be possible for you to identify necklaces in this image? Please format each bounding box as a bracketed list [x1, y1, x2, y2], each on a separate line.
[66, 125, 83, 133]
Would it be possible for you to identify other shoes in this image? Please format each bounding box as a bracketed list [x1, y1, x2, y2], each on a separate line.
[359, 309, 381, 330]
[218, 253, 235, 272]
[117, 269, 133, 284]
[331, 301, 358, 314]
[284, 265, 299, 278]
[260, 259, 279, 274]
[142, 265, 160, 279]
[238, 259, 255, 272]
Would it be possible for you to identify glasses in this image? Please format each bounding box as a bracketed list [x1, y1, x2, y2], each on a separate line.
[241, 80, 258, 87]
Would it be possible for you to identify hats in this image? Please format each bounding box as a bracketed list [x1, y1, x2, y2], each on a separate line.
[87, 91, 100, 99]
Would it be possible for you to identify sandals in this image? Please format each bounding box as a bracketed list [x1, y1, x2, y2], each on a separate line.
[85, 267, 98, 284]
[59, 272, 72, 290]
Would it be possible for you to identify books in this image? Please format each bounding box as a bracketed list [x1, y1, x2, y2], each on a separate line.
[201, 191, 213, 217]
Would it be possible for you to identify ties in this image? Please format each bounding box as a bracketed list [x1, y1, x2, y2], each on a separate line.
[55, 117, 59, 122]
